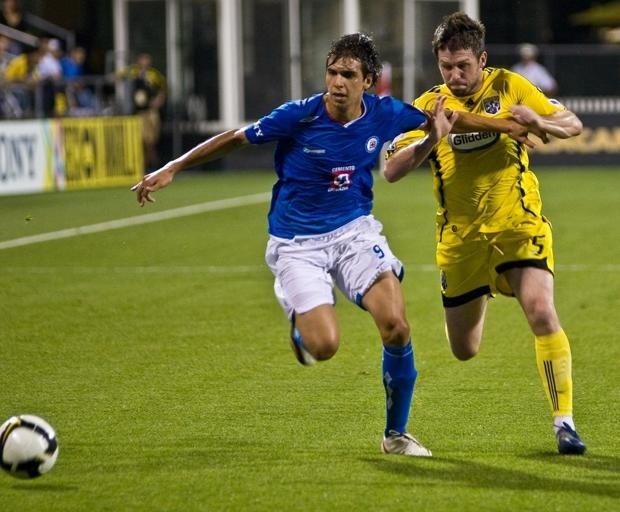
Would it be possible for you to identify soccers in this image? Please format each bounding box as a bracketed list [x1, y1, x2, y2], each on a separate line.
[0, 414, 60, 481]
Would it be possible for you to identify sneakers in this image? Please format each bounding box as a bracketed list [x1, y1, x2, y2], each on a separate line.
[381, 433, 433, 457]
[290, 316, 317, 366]
[557, 425, 586, 456]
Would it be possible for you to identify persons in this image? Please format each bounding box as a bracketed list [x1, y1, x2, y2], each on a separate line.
[380, 12, 589, 457]
[0, 0, 166, 169]
[509, 45, 559, 96]
[130, 33, 549, 457]
